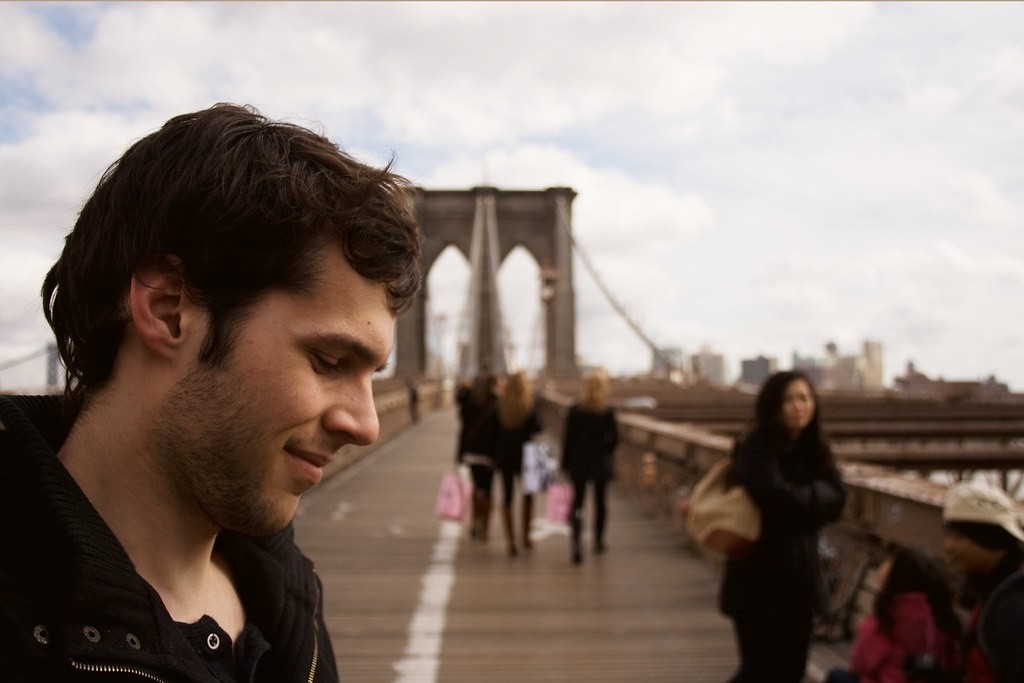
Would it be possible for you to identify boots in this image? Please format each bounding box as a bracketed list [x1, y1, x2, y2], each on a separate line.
[470, 488, 495, 540]
[503, 502, 534, 558]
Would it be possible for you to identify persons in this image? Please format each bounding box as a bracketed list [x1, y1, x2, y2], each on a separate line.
[825, 481, 1024, 683]
[719, 372, 847, 683]
[487, 371, 548, 557]
[0, 102, 421, 683]
[561, 370, 619, 566]
[407, 372, 509, 543]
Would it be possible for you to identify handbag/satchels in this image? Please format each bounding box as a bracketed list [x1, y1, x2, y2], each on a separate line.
[518, 437, 560, 496]
[686, 458, 759, 553]
[547, 474, 573, 523]
[434, 463, 473, 520]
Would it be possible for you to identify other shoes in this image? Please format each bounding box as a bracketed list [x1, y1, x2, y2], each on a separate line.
[595, 539, 608, 552]
[571, 548, 582, 564]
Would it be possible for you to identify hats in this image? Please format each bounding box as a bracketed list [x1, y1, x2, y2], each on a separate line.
[943, 481, 1024, 541]
